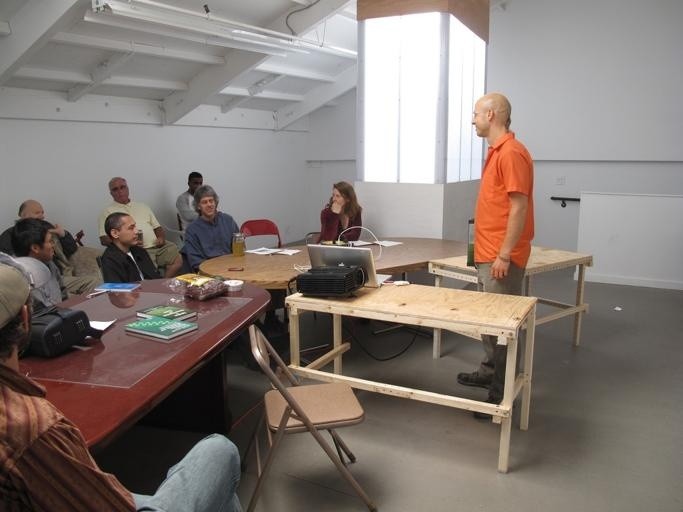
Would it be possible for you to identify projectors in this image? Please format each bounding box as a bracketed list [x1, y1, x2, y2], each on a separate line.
[297, 266, 369, 298]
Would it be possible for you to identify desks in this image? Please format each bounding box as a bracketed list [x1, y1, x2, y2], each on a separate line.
[18, 276, 274, 455]
[197, 236, 467, 350]
[284, 279, 539, 475]
[428, 244, 593, 360]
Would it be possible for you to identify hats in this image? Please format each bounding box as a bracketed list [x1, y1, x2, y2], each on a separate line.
[0, 251, 52, 331]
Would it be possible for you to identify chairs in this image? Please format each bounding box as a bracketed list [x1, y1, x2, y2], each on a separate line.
[239, 219, 281, 248]
[305, 232, 321, 245]
[242, 323, 378, 512]
[158, 223, 186, 278]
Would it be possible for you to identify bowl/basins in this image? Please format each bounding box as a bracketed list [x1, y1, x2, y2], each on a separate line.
[223, 280, 243, 292]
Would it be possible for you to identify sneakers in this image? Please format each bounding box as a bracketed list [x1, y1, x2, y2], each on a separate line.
[474, 400, 516, 419]
[457, 372, 491, 389]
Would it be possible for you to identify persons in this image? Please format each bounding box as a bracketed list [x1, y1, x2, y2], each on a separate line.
[320, 181, 361, 242]
[177, 172, 203, 229]
[0, 253, 242, 512]
[0, 201, 99, 294]
[10, 218, 62, 308]
[102, 213, 161, 281]
[98, 178, 183, 278]
[185, 185, 281, 329]
[457, 93, 535, 419]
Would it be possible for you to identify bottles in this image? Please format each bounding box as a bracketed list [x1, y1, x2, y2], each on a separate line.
[231, 232, 244, 257]
[136, 229, 144, 245]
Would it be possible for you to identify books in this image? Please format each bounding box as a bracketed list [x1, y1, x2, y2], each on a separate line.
[136, 305, 197, 321]
[94, 283, 141, 292]
[124, 316, 198, 339]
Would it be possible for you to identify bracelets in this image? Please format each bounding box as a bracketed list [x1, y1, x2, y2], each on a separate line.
[498, 255, 510, 262]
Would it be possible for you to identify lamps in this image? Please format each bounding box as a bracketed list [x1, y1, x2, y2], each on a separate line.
[83, 0, 358, 61]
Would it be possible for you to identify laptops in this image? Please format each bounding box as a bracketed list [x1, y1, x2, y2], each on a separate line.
[306, 243, 392, 289]
